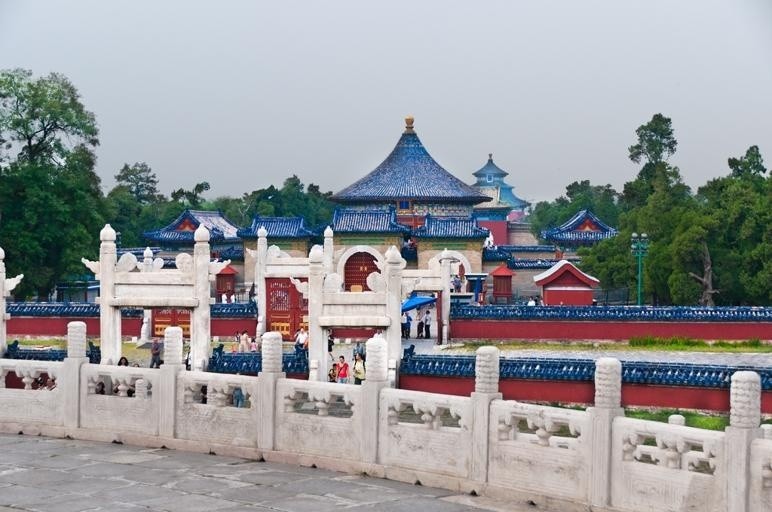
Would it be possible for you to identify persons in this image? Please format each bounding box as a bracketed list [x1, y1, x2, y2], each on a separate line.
[449, 274, 463, 293]
[400, 304, 432, 340]
[525, 295, 542, 307]
[35, 355, 153, 397]
[148, 339, 161, 368]
[184, 323, 368, 409]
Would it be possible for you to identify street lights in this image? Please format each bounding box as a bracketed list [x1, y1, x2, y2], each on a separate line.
[629, 231, 651, 305]
